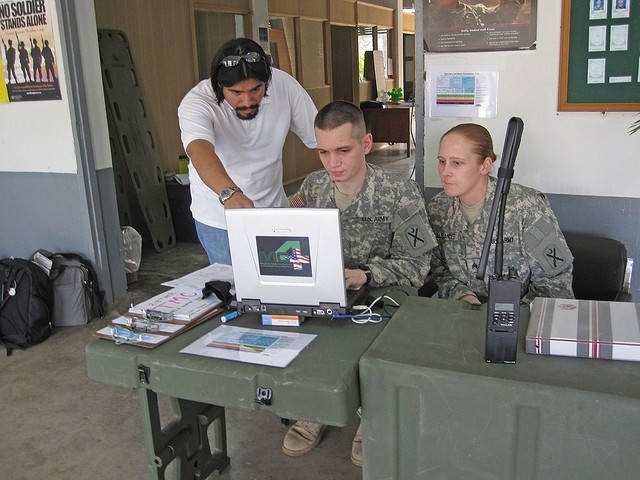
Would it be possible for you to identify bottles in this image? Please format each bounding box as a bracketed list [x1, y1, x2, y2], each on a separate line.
[383, 92, 388, 109]
[179, 155, 189, 174]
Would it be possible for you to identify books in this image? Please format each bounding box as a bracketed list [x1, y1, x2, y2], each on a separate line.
[129, 288, 223, 321]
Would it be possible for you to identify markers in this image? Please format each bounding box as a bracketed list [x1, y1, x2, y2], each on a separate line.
[221, 309, 242, 322]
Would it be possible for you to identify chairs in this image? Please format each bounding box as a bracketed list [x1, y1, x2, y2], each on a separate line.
[418, 230, 633, 302]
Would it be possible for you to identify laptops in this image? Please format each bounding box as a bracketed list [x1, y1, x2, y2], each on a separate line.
[224, 207, 369, 318]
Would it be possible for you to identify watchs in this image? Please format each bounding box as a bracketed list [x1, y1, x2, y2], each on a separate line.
[219, 186, 242, 203]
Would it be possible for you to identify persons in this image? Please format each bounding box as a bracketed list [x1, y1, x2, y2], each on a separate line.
[426, 124, 576, 303]
[5, 39, 18, 84]
[42, 40, 57, 82]
[281, 100, 440, 467]
[17, 41, 33, 82]
[177, 38, 319, 264]
[30, 38, 42, 83]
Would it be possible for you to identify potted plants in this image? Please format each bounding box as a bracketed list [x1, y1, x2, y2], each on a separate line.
[388, 87, 402, 104]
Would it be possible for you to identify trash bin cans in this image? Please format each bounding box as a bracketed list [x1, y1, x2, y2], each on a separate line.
[120, 226, 142, 286]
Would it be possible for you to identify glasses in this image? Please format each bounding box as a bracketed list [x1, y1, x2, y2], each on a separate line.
[218, 52, 265, 68]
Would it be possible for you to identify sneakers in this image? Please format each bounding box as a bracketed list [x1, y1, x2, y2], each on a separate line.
[350, 422, 363, 466]
[281, 421, 327, 457]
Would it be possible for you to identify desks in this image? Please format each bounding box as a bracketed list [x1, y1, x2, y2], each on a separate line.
[360, 107, 413, 158]
[359, 295, 640, 480]
[85, 265, 417, 479]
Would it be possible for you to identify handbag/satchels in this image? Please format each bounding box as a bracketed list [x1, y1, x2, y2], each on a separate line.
[1, 258, 54, 356]
[27, 249, 105, 327]
